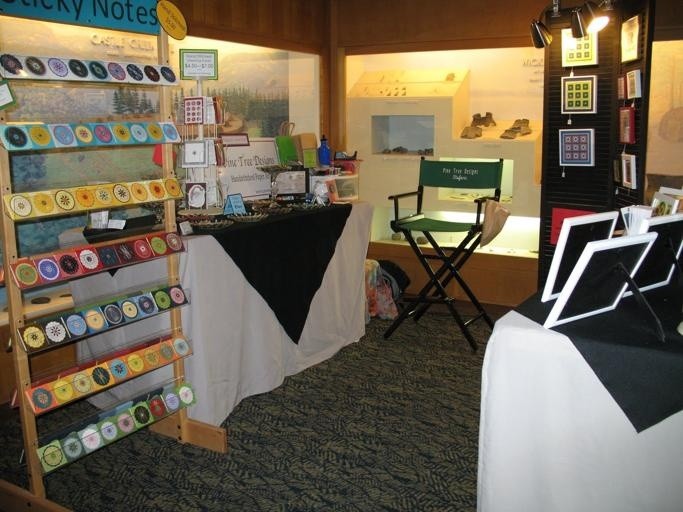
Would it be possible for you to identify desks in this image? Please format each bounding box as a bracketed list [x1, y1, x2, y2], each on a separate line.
[57, 199, 372, 425]
[476, 257, 683, 512]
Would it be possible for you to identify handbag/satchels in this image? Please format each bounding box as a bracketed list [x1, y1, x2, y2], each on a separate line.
[275, 120, 297, 163]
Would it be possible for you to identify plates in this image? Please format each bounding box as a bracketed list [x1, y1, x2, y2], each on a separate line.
[182, 193, 328, 231]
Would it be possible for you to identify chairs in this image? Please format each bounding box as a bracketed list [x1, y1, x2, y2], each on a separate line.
[382, 155, 506, 350]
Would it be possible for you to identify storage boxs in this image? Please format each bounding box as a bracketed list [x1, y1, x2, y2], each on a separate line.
[309, 176, 359, 201]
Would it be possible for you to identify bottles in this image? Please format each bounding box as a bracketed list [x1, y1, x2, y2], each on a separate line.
[319, 136, 329, 165]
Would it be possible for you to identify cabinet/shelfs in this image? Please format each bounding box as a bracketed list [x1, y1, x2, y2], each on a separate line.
[0, 0, 226, 512]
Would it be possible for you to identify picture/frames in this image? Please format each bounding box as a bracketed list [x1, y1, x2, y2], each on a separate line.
[621, 13, 641, 64]
[270, 168, 309, 198]
[627, 69, 641, 99]
[540, 185, 683, 344]
[619, 107, 636, 144]
[617, 76, 627, 100]
[559, 128, 596, 168]
[611, 153, 637, 190]
[561, 28, 598, 67]
[560, 75, 597, 115]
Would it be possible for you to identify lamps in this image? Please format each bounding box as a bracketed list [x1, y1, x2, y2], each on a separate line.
[580, 0, 612, 35]
[527, 0, 560, 49]
[571, 6, 588, 38]
